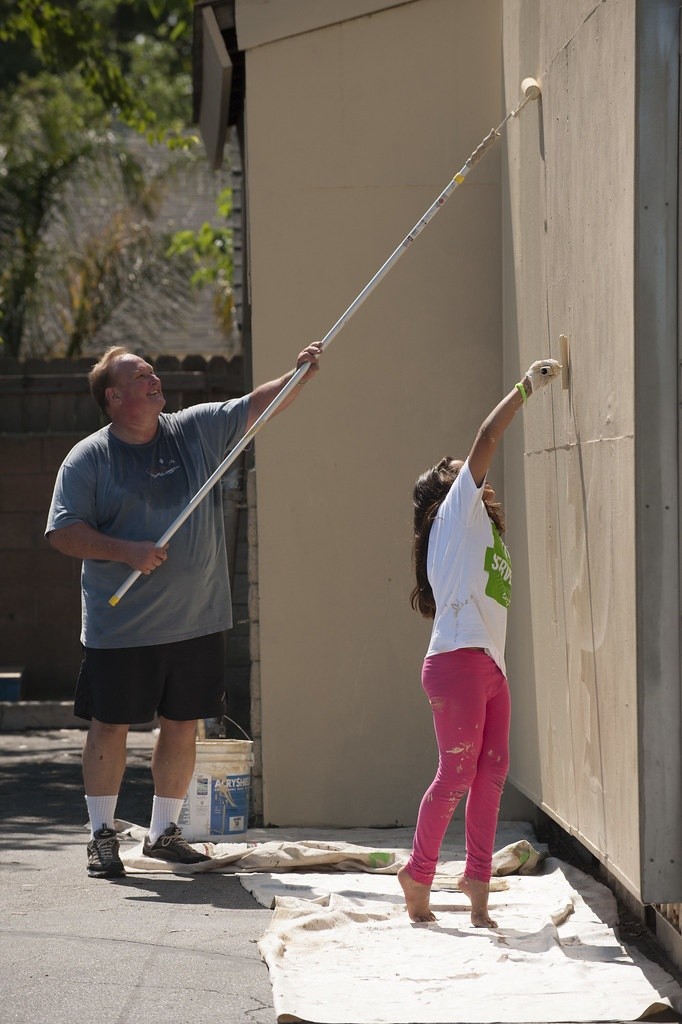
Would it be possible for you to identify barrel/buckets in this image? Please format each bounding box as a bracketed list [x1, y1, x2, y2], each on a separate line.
[176, 740, 253, 842]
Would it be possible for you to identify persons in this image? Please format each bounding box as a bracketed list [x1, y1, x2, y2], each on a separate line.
[399, 360, 564, 928]
[44, 341, 325, 877]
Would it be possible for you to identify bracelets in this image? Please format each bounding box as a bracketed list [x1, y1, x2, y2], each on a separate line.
[292, 368, 308, 384]
[516, 384, 529, 406]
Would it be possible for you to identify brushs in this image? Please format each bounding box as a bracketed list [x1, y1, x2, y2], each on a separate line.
[542, 334, 571, 392]
[105, 77, 541, 607]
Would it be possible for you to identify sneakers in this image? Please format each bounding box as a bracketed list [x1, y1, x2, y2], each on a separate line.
[142, 822, 213, 864]
[86, 823, 127, 878]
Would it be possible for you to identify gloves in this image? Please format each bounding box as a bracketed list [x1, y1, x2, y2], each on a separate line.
[525, 359, 562, 393]
